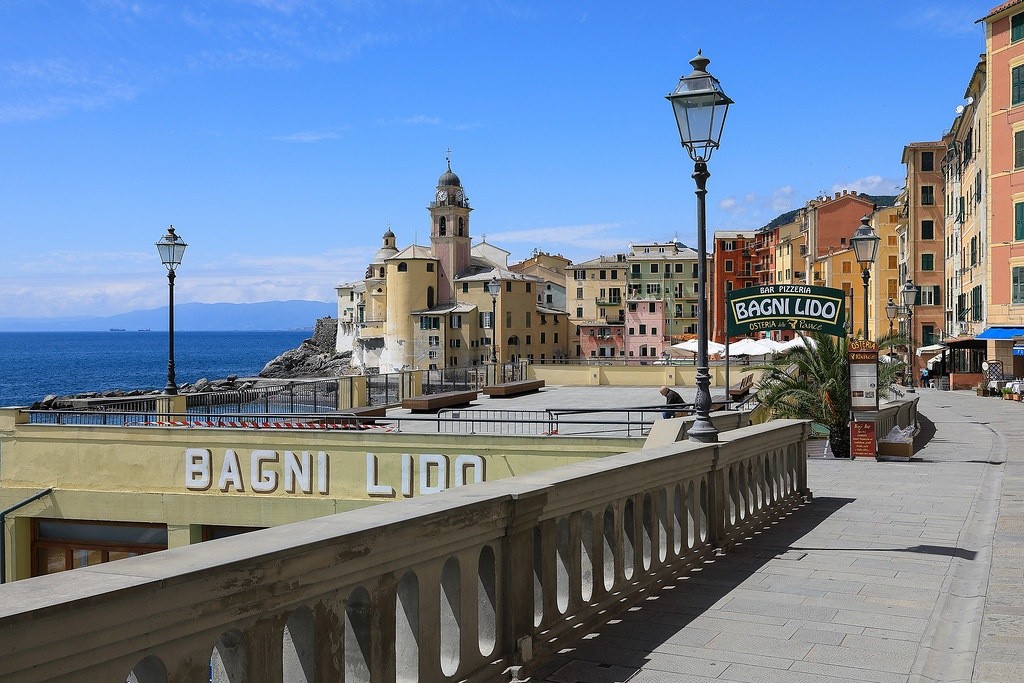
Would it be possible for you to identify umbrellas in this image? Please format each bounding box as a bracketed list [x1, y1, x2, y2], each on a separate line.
[671, 336, 817, 365]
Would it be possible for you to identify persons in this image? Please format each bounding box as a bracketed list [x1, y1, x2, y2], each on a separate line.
[921, 367, 929, 388]
[659, 386, 686, 419]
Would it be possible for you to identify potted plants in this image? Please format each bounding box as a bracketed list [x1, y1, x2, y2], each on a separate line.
[1000, 387, 1013, 400]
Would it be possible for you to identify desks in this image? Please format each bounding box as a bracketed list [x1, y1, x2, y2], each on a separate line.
[986, 380, 1024, 403]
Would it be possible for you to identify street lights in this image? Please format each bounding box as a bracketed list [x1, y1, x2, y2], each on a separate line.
[901, 275, 919, 393]
[487, 275, 501, 363]
[850, 214, 882, 340]
[156, 224, 188, 395]
[885, 298, 898, 366]
[666, 49, 737, 445]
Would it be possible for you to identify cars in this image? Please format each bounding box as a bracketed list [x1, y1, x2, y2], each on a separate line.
[652, 358, 669, 365]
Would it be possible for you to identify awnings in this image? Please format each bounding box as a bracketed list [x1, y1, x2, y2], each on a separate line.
[927, 349, 950, 370]
[916, 344, 947, 357]
[974, 327, 1024, 356]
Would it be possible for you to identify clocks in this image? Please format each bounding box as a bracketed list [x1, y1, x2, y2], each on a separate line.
[455, 190, 463, 202]
[437, 189, 447, 202]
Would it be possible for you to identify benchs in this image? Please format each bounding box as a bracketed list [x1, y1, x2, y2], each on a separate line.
[284, 405, 387, 426]
[483, 379, 545, 399]
[401, 390, 478, 414]
[702, 393, 727, 412]
[728, 373, 754, 402]
[667, 403, 690, 417]
